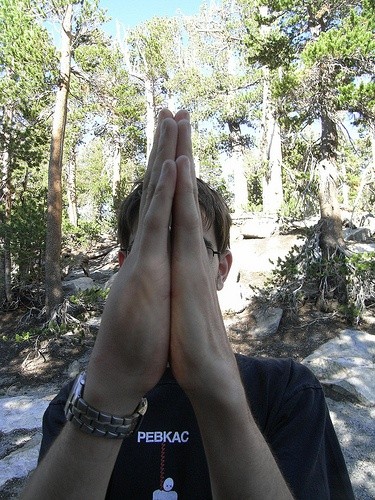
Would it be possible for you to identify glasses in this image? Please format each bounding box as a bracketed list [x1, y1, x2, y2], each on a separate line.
[121, 244, 223, 266]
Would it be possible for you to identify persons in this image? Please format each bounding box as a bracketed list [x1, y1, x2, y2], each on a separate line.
[17, 109, 355, 500]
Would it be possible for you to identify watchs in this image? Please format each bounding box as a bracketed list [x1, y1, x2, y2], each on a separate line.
[64, 372, 149, 440]
